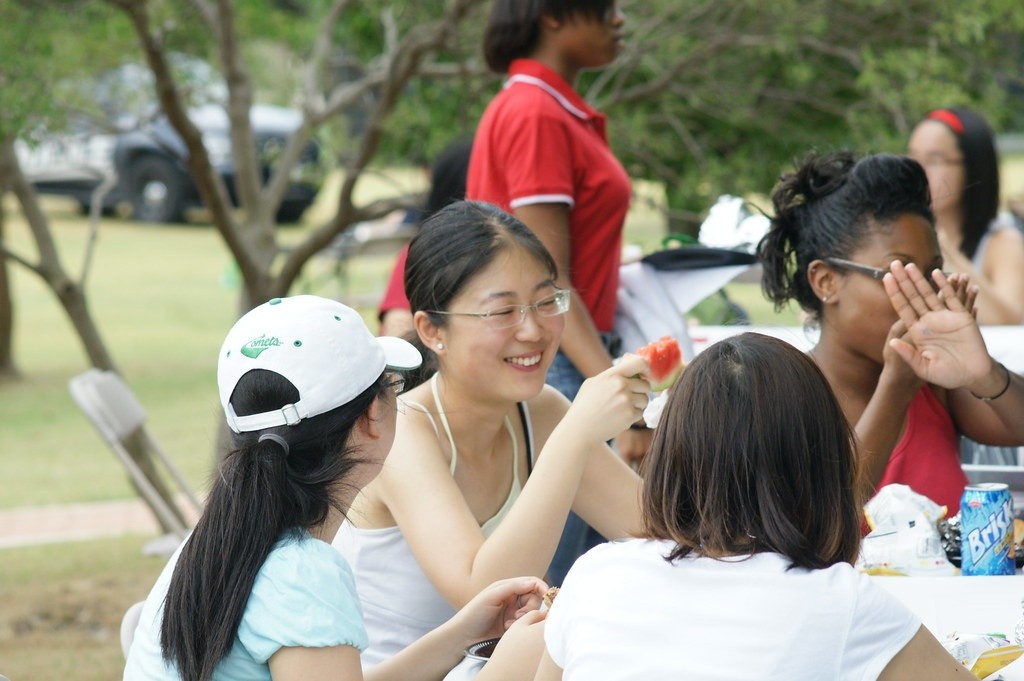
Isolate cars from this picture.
[15,49,322,223]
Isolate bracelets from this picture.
[631,423,647,432]
[970,362,1010,401]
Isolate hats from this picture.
[216,293,422,434]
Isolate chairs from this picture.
[67,367,201,542]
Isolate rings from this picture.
[517,595,522,608]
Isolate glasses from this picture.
[425,286,571,329]
[825,257,952,287]
[379,371,405,394]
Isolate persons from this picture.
[906,108,1024,327]
[466,0,651,587]
[762,153,1024,536]
[377,130,478,336]
[535,332,978,681]
[332,201,645,672]
[122,295,550,681]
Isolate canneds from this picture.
[959,483,1015,577]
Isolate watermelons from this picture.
[613,335,687,392]
